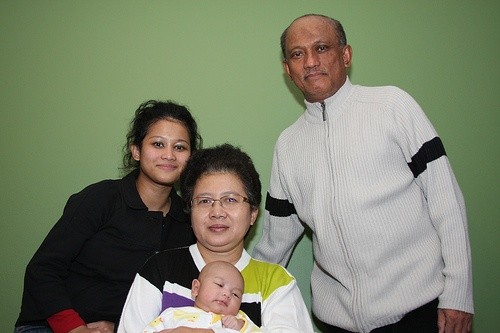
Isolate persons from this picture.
[143,261,259,332]
[115,143,314,333]
[249,14,474,333]
[13,99,202,333]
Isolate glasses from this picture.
[190,195,253,207]
